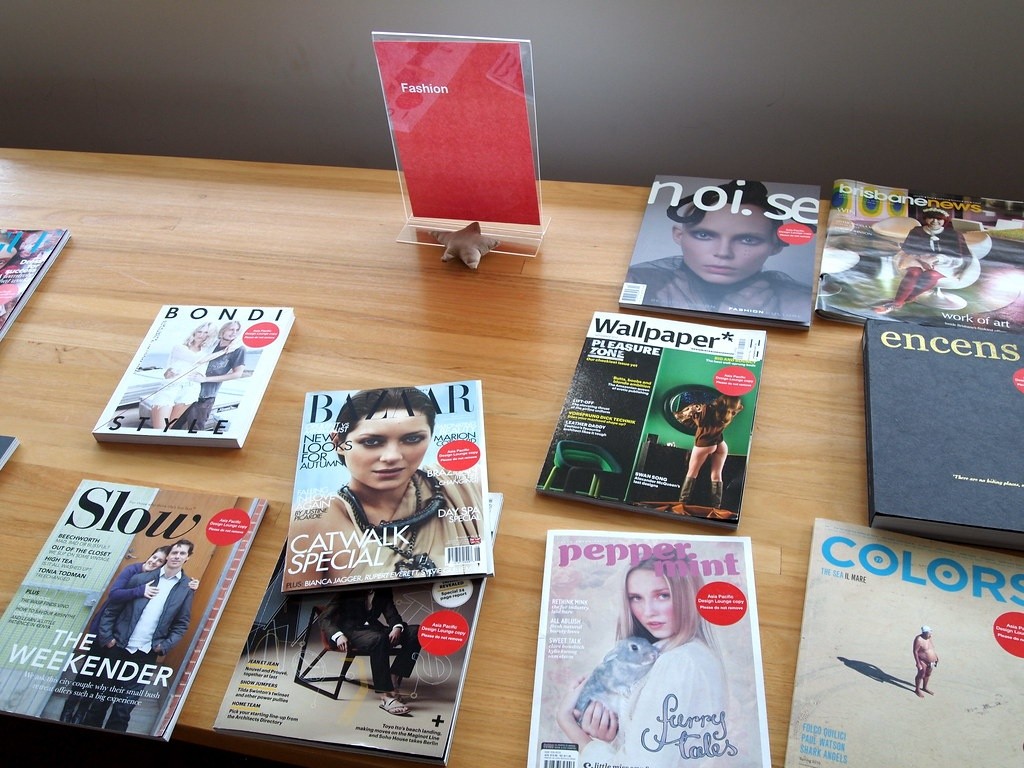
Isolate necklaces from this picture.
[338,474,440,580]
[345,468,443,535]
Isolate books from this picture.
[280,379,497,593]
[535,311,766,528]
[816,175,1024,329]
[783,515,1024,768]
[0,477,270,743]
[212,486,504,763]
[616,175,820,331]
[522,529,775,768]
[0,227,72,343]
[0,436,21,470]
[865,320,1024,552]
[91,304,296,449]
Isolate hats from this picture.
[921,625,932,633]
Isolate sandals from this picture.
[379,697,411,715]
[392,694,406,704]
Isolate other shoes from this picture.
[889,296,917,304]
[72,712,84,724]
[59,703,76,723]
[873,301,902,314]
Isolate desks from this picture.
[0,149,1024,767]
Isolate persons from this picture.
[618,178,812,325]
[555,549,728,768]
[139,319,246,431]
[318,589,422,715]
[284,384,482,589]
[0,241,17,271]
[913,625,939,698]
[59,537,199,732]
[871,207,960,316]
[679,401,729,509]
